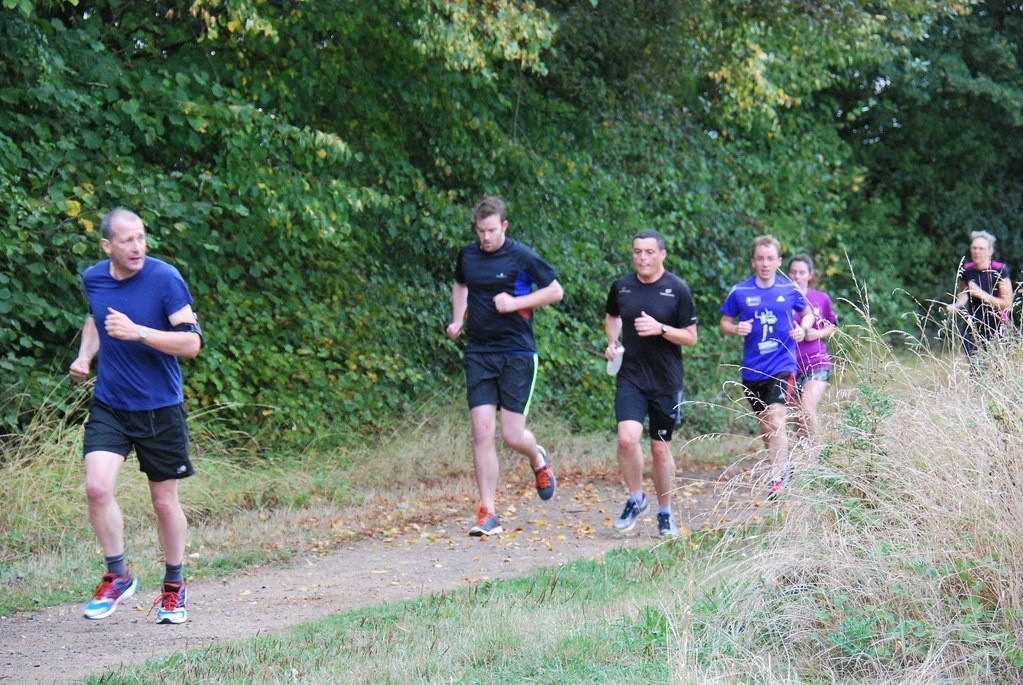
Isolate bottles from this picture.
[606,341,625,376]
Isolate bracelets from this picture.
[800,326,808,338]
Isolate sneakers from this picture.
[469,511,503,537]
[85,567,138,620]
[155,582,188,625]
[768,480,783,500]
[658,512,674,537]
[530,445,556,501]
[616,493,649,533]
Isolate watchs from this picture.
[138,326,148,343]
[659,321,668,337]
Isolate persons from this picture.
[71,207,205,624]
[718,235,813,502]
[448,196,565,537]
[784,253,835,449]
[946,231,1013,386]
[602,230,698,537]
